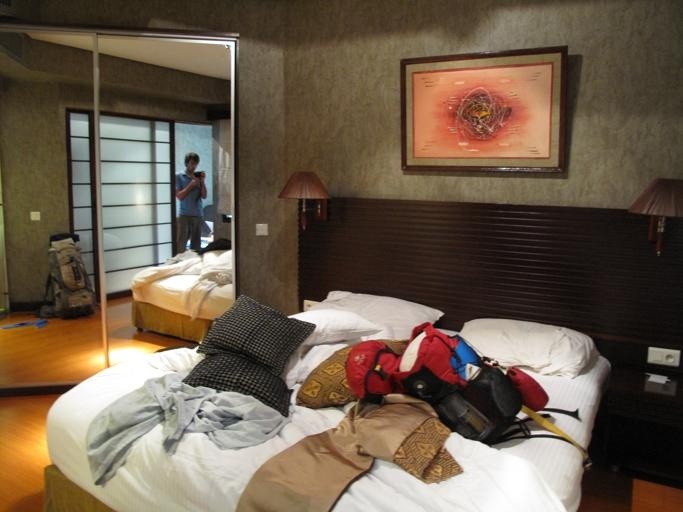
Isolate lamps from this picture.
[276,171,330,232]
[628,174,681,263]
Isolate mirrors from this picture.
[0,22,237,400]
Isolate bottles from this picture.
[439,391,493,441]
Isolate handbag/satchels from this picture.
[345,341,399,403]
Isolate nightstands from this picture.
[602,362,682,492]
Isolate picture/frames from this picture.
[397,42,567,175]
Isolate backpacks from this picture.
[391,322,592,469]
[40,239,96,320]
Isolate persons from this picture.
[175,152,207,253]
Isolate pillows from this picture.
[181,356,295,419]
[196,292,317,379]
[304,290,446,342]
[458,318,596,380]
[293,340,408,411]
[287,305,385,343]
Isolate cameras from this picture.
[194,172,202,177]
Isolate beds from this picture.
[126,247,234,346]
[41,193,640,512]
